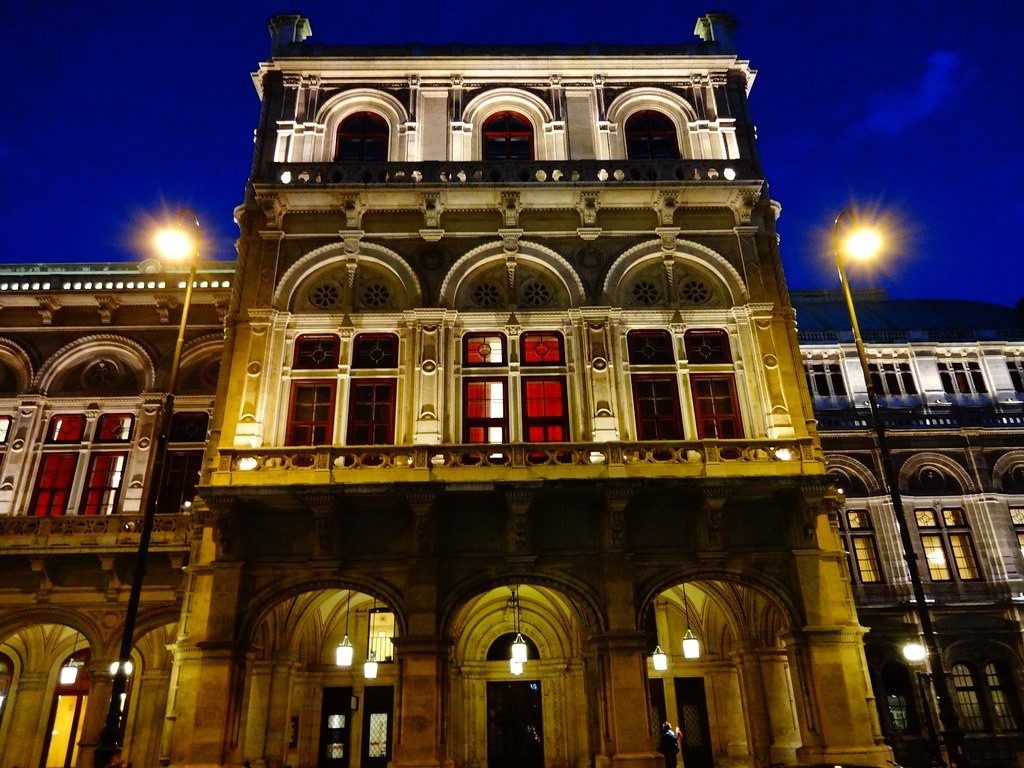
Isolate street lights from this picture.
[89,208,202,768]
[832,204,972,768]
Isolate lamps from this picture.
[510,593,524,676]
[336,590,354,667]
[682,583,700,660]
[652,643,668,671]
[511,584,527,664]
[60,632,79,685]
[363,599,379,680]
